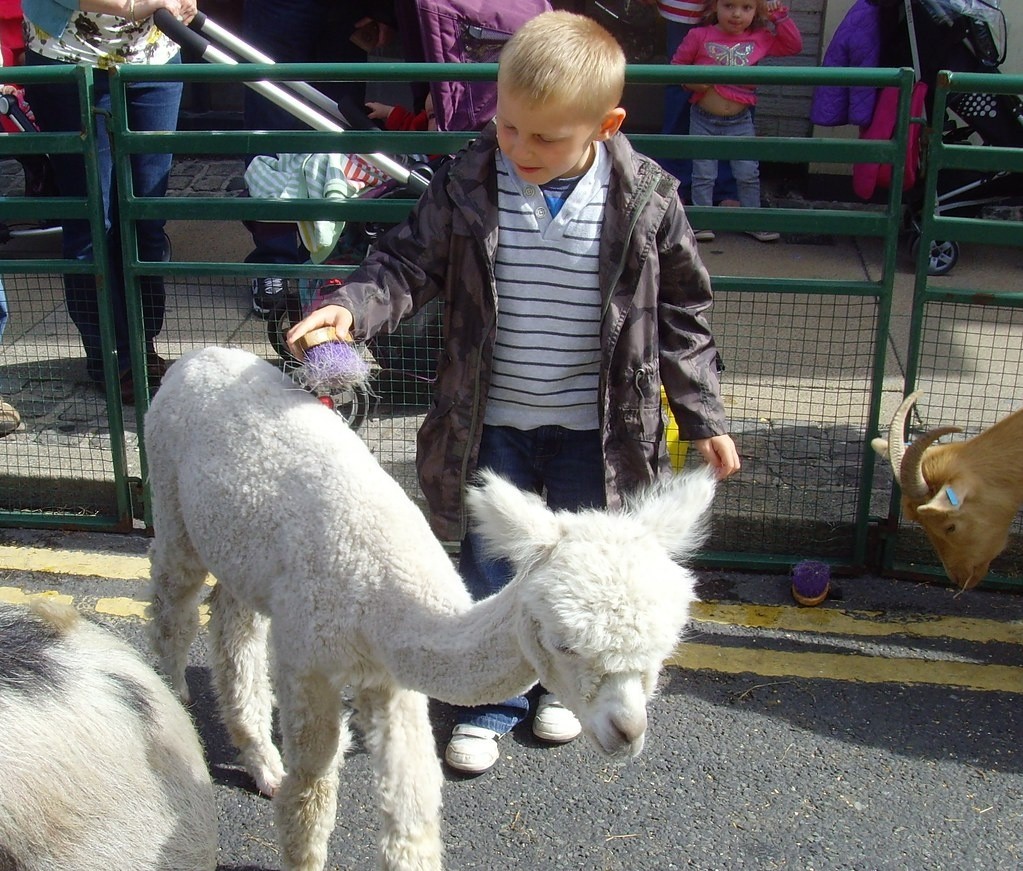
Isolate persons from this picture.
[0,0,803,435]
[286,10,741,773]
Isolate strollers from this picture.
[869,0,1022,273]
[151,5,473,432]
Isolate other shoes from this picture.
[692,229,714,241]
[99,371,136,404]
[147,359,167,386]
[746,227,781,240]
[0,397,21,432]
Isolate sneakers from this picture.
[445,721,502,773]
[533,692,583,743]
[251,272,290,316]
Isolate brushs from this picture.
[299,327,371,387]
[791,560,830,607]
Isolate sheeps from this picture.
[1,587,229,870]
[871,390,1023,593]
